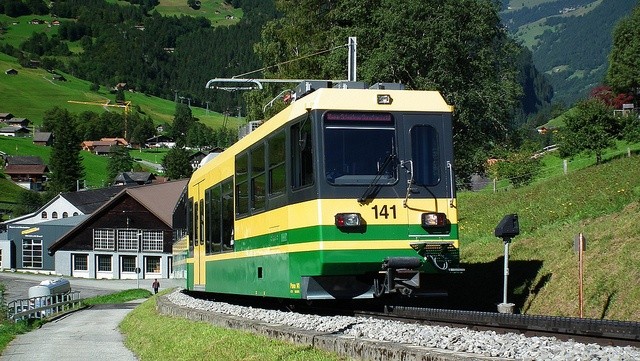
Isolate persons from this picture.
[152,279,159,295]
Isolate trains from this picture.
[172,80,466,313]
